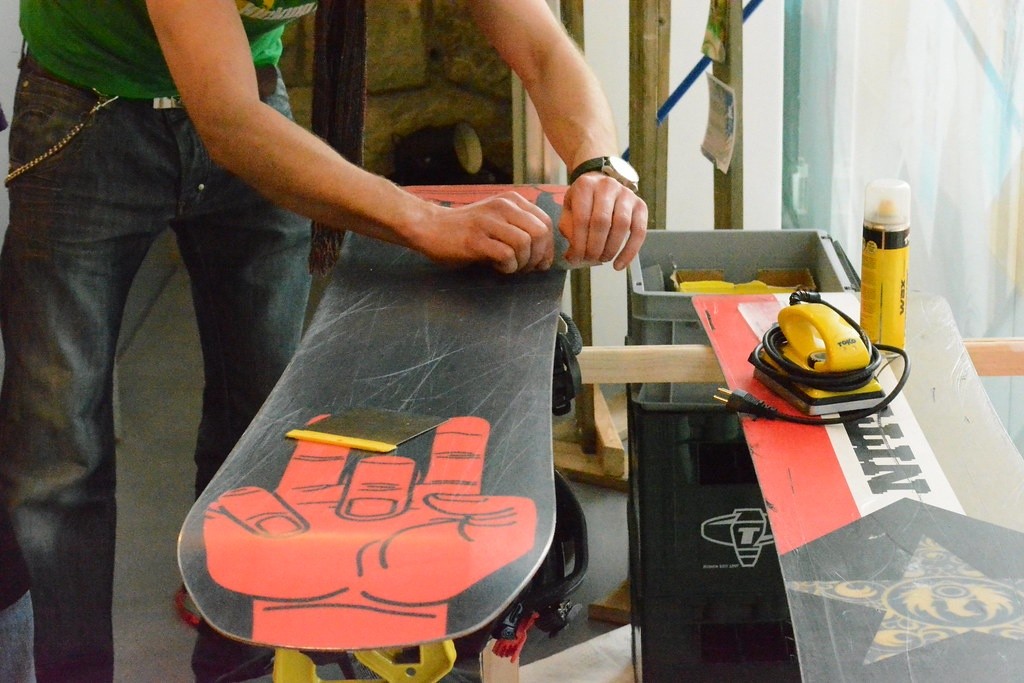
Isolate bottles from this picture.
[859,180,911,360]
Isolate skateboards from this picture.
[177,183,571,652]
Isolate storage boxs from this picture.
[622,229,862,412]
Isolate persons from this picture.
[0,0,649,683]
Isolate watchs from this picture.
[569,155,641,194]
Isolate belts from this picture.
[24,46,278,108]
